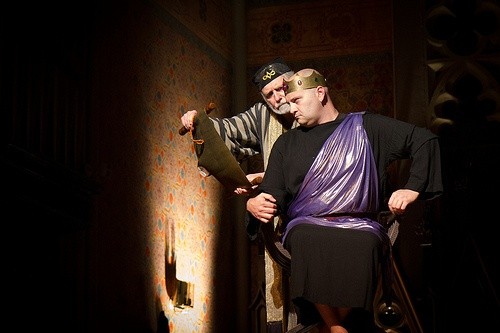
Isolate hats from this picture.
[249,56,292,93]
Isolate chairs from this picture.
[263,196,423,333]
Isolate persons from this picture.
[245,67,442,333]
[180,65,296,331]
